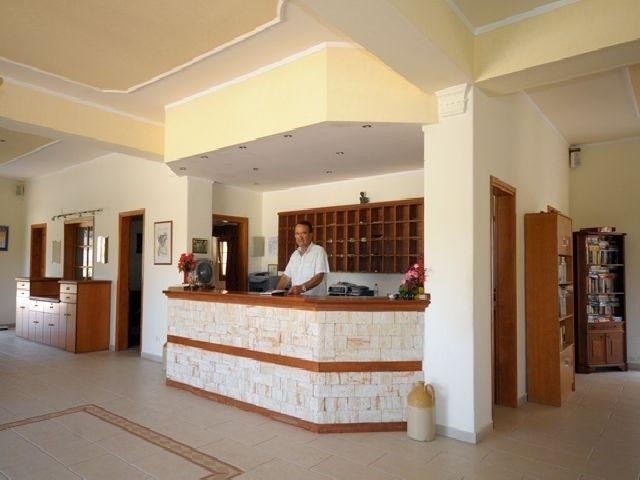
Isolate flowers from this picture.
[400,256,424,300]
[178,254,196,271]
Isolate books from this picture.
[561,324,565,351]
[588,266,617,292]
[558,285,567,317]
[588,295,622,322]
[587,235,618,264]
[557,256,566,282]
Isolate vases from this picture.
[184,273,189,283]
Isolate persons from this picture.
[274,220,330,296]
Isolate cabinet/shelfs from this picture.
[525,211,574,404]
[573,230,628,374]
[28,296,60,347]
[59,281,110,352]
[15,277,62,337]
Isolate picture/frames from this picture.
[0,225,8,251]
[154,221,173,265]
[191,238,209,259]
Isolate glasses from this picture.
[295,232,310,235]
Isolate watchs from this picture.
[301,284,306,291]
